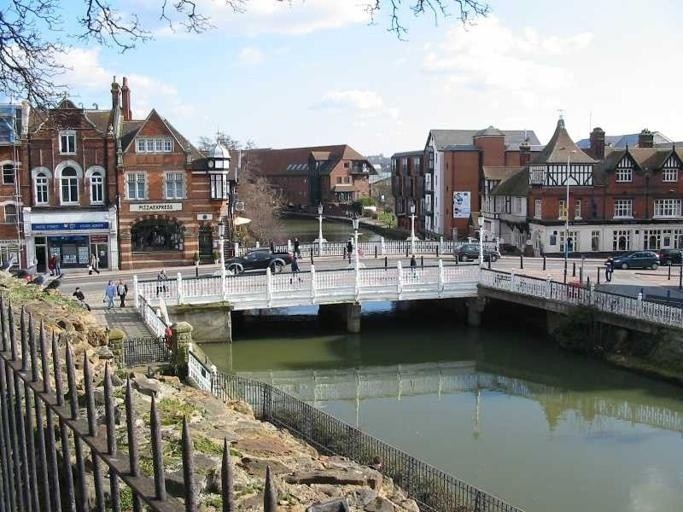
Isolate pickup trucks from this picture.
[223,248,295,273]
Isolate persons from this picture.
[293,237,303,259]
[115,279,128,308]
[604,255,614,282]
[156,268,169,297]
[103,279,117,309]
[87,253,100,275]
[48,255,55,276]
[54,253,60,276]
[345,242,353,264]
[410,254,418,279]
[288,257,303,285]
[72,287,85,302]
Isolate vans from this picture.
[602,251,663,272]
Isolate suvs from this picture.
[656,247,683,267]
[453,243,501,262]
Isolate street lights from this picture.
[345,210,365,296]
[472,387,481,442]
[408,199,420,253]
[564,147,579,278]
[312,201,329,257]
[475,211,486,269]
